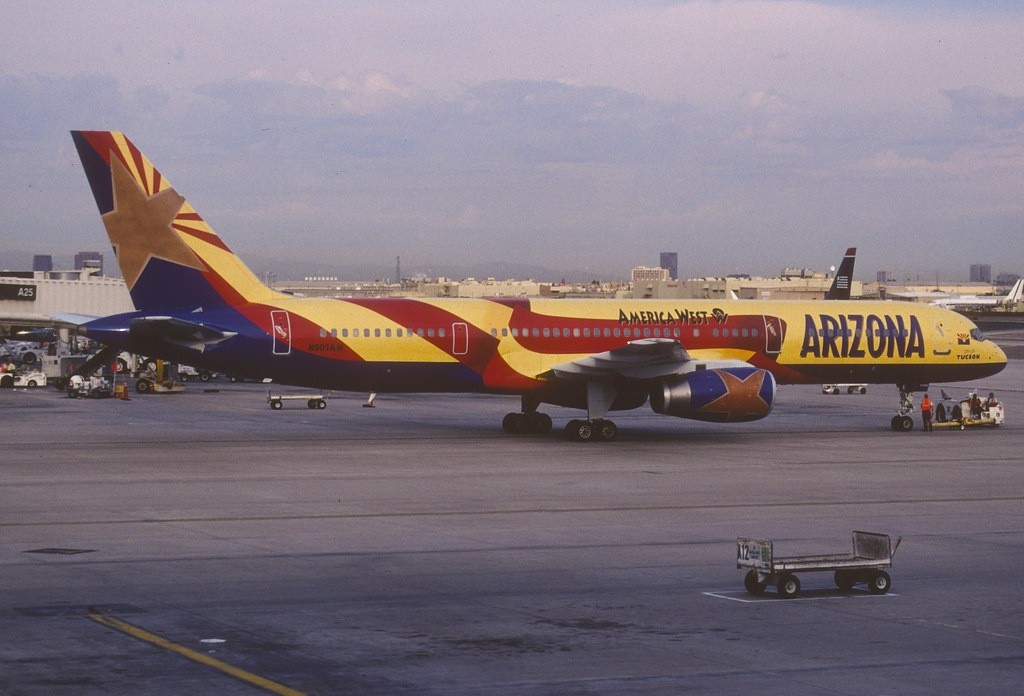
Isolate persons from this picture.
[921,393,934,432]
[969,394,984,419]
[985,392,998,411]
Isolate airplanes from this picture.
[45,126,1015,443]
[924,278,1024,316]
